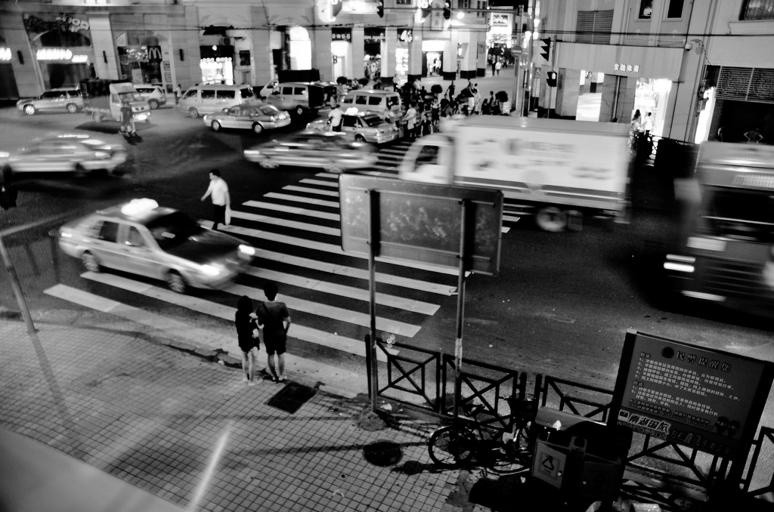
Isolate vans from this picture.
[175,84,256,120]
[265,82,323,116]
[337,89,401,122]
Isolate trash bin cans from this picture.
[528,406,633,502]
[654,136,700,182]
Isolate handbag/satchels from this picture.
[225,205,232,225]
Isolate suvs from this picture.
[132,84,166,110]
[15,87,83,116]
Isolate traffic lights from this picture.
[0,163,18,212]
[546,72,556,87]
[540,37,551,61]
[442,0,452,20]
[376,1,384,19]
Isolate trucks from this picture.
[660,141,773,322]
[78,78,151,126]
[259,69,320,101]
[397,115,633,234]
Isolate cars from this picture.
[202,99,291,135]
[242,128,376,175]
[0,129,127,188]
[305,107,399,144]
[57,198,256,294]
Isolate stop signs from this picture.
[511,45,522,58]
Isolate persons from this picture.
[716,126,728,142]
[392,72,482,140]
[255,282,291,381]
[120,100,136,134]
[491,55,507,76]
[175,83,182,104]
[632,109,641,127]
[743,127,763,144]
[365,58,381,78]
[486,90,495,116]
[338,76,360,103]
[234,294,264,386]
[199,168,230,231]
[328,104,343,131]
[642,111,652,135]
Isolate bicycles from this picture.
[427,392,538,473]
